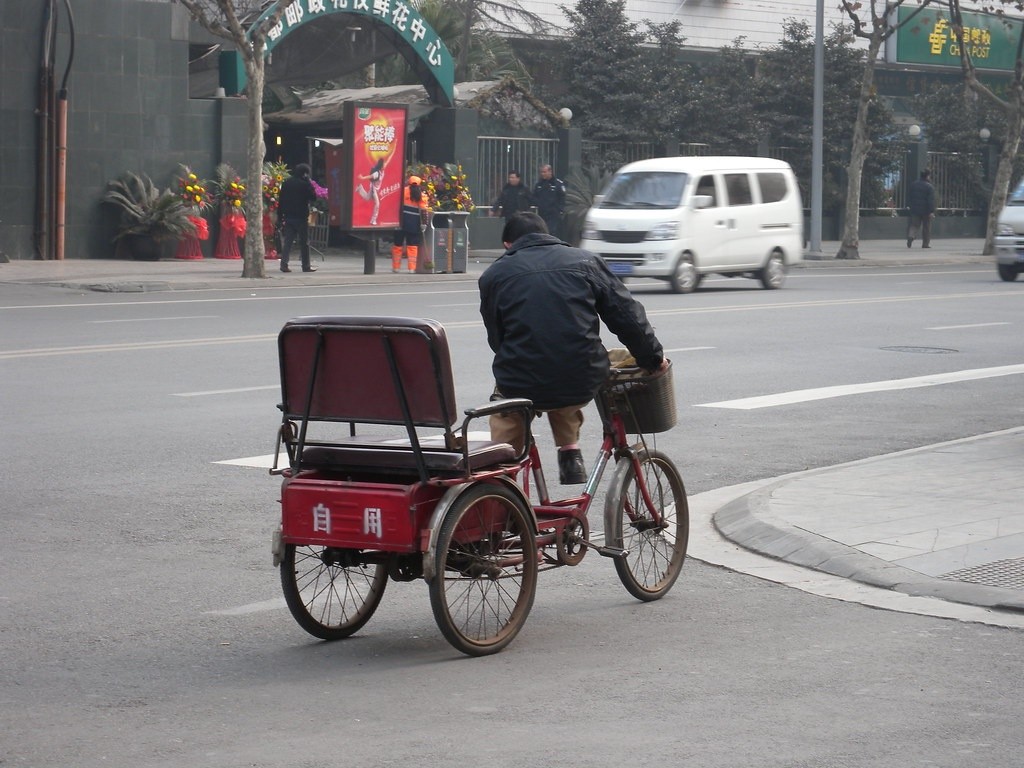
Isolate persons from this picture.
[532,165,566,238]
[392,175,428,274]
[489,171,532,224]
[355,139,398,225]
[275,163,317,273]
[906,169,935,248]
[478,212,667,485]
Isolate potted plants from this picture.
[101,169,200,261]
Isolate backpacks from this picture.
[369,168,380,182]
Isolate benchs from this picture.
[277,316,515,471]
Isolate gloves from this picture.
[420,224,427,233]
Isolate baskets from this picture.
[594,358,677,434]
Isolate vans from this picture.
[580,155,804,292]
[993,175,1024,281]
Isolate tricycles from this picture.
[269,315,690,657]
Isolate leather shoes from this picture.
[558,449,587,484]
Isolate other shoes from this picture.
[921,245,932,248]
[280,267,291,272]
[392,268,399,273]
[409,270,417,274]
[355,184,362,192]
[370,221,377,225]
[907,237,913,248]
[303,268,317,272]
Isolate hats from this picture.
[410,176,421,185]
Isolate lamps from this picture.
[979,127,991,143]
[558,107,574,127]
[908,125,921,141]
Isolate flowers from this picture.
[211,162,246,207]
[311,180,329,199]
[259,160,291,208]
[404,161,476,214]
[174,161,217,212]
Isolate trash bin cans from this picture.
[415,211,470,274]
